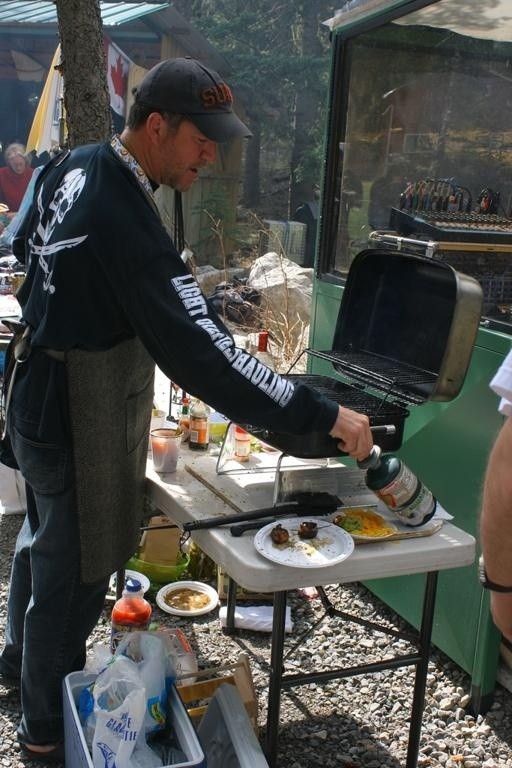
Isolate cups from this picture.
[149,426,184,472]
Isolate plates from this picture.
[104,568,151,600]
[327,511,398,542]
[253,516,357,570]
[156,579,220,617]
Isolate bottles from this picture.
[252,331,277,376]
[358,444,437,527]
[234,426,250,461]
[110,578,152,659]
[189,399,210,451]
[178,397,191,441]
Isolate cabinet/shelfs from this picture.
[364,228,512,331]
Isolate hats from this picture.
[134,58,253,144]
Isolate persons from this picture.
[0,54,375,761]
[480,347,512,667]
[1,142,34,223]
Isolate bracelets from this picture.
[478,553,511,593]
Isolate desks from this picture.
[121,366,481,762]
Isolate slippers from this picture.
[19,740,65,762]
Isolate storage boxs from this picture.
[59,668,208,767]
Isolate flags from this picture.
[106,38,131,117]
